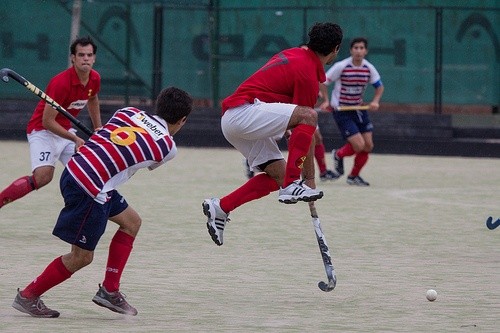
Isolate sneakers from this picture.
[202,198,230,246]
[92,284,137,316]
[244,158,254,179]
[278,179,324,204]
[333,149,344,175]
[12,288,60,318]
[346,176,370,187]
[319,169,340,182]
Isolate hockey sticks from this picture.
[308,200,337,293]
[486,216,500,231]
[313,105,371,113]
[0,67,93,137]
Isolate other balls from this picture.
[425,289,438,302]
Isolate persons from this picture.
[319,38,384,187]
[202,22,343,246]
[0,37,103,208]
[12,87,192,318]
